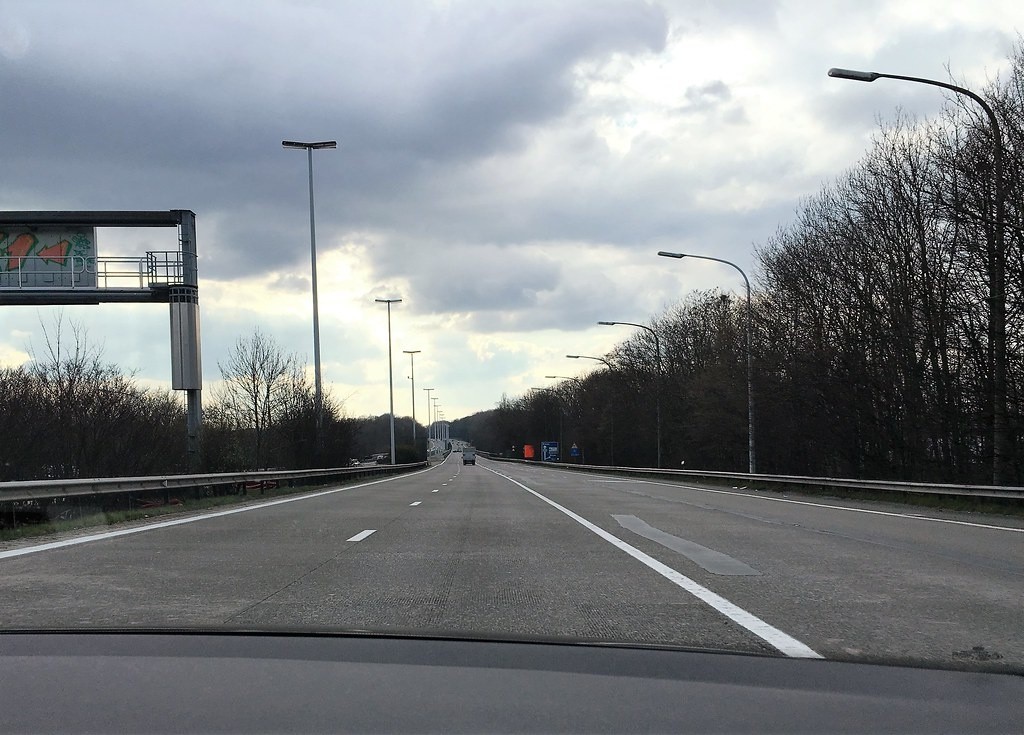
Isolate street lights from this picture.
[402,349,421,440]
[566,354,614,465]
[375,296,403,465]
[597,321,662,469]
[658,249,758,472]
[422,387,448,465]
[828,66,1012,485]
[530,375,587,465]
[280,133,339,466]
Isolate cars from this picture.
[346,451,390,466]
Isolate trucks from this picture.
[461,447,478,465]
